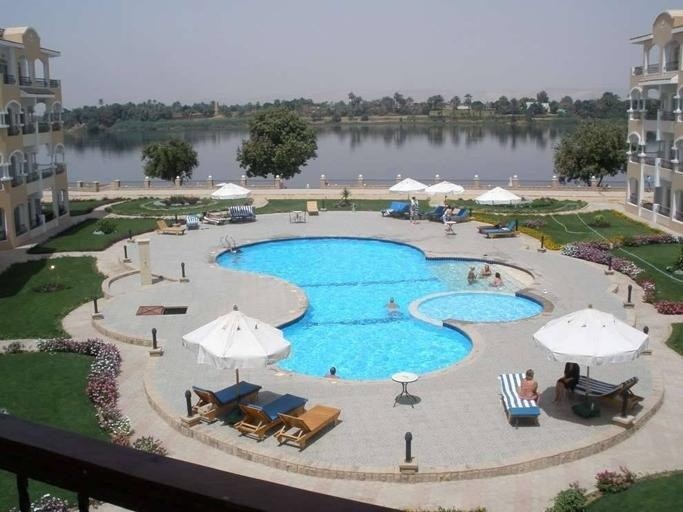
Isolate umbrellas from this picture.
[426,180,465,206]
[387,177,428,201]
[180,303,292,424]
[209,182,251,207]
[473,186,521,229]
[530,307,650,419]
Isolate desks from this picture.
[290,210,305,224]
[389,372,420,409]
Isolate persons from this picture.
[438,203,455,226]
[198,209,225,226]
[551,362,579,407]
[490,272,502,288]
[408,196,419,224]
[517,368,540,403]
[479,265,491,275]
[382,297,401,312]
[467,265,478,285]
[324,367,341,379]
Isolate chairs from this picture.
[498,373,541,430]
[379,200,518,240]
[153,203,256,236]
[188,377,341,452]
[559,374,644,419]
[306,200,318,216]
